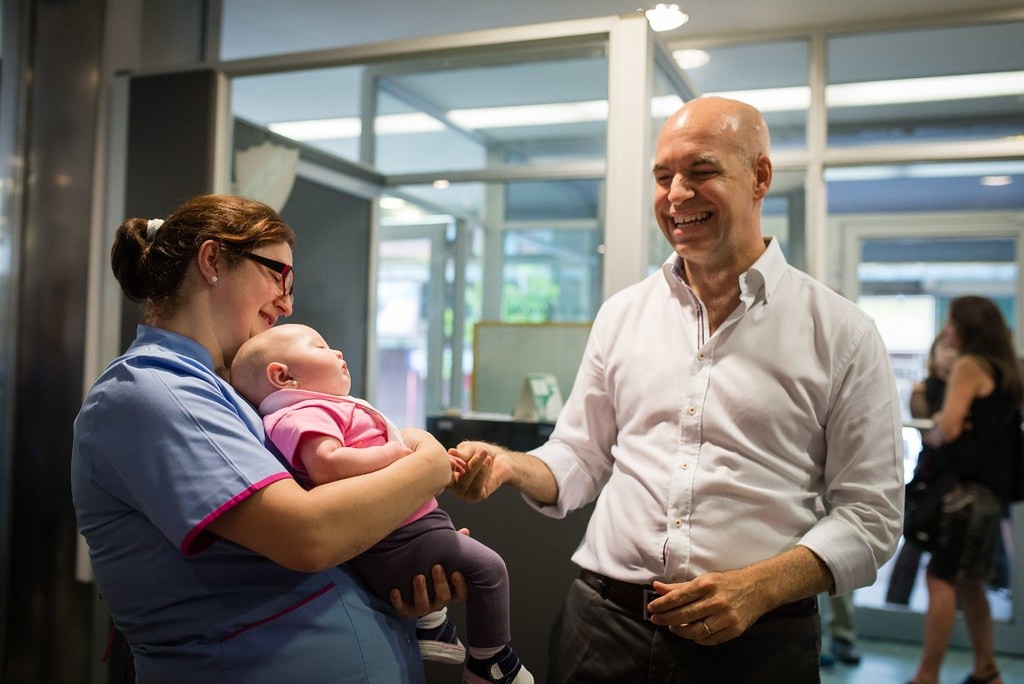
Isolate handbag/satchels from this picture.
[904,471,972,556]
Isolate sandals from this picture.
[962,671,1003,684]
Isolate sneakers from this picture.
[462,645,535,684]
[415,615,466,664]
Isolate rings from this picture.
[701,620,712,636]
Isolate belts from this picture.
[579,569,818,633]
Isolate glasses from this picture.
[223,238,295,297]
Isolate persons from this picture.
[228,324,534,684]
[442,96,905,684]
[905,293,1024,684]
[883,332,968,613]
[68,193,470,684]
